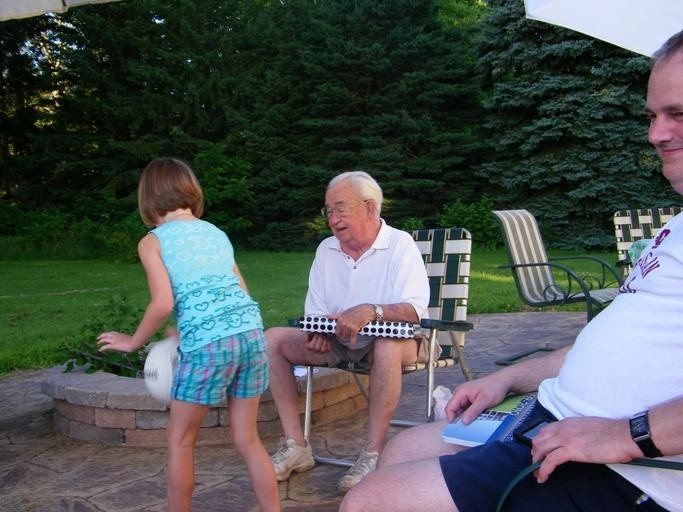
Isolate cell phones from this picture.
[513,414,553,448]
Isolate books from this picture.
[442,389,540,448]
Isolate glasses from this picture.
[321,199,366,218]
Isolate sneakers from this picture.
[336,447,379,493]
[270,436,315,481]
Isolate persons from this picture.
[265,170,443,491]
[96,158,282,511]
[341,31,682,512]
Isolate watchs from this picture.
[370,302,384,323]
[630,410,662,459]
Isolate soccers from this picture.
[144,336,181,407]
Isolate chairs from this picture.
[490,208,623,365]
[613,208,683,282]
[493,458,682,511]
[288,227,472,468]
